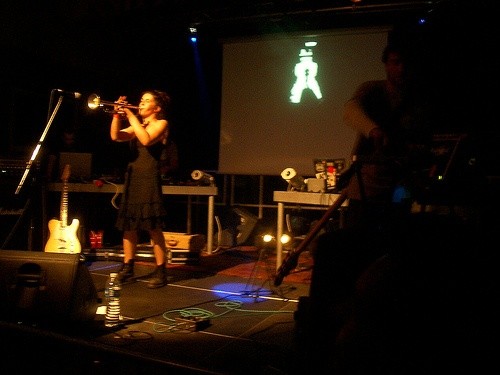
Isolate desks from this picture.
[42,183,219,256]
[273,191,432,273]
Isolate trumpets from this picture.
[87,94,143,120]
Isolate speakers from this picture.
[0,250,97,334]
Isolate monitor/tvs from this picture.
[60,153,92,179]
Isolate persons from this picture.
[156,141,179,225]
[43,129,86,218]
[344,40,430,238]
[110,90,173,289]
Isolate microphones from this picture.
[53,88,81,99]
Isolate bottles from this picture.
[104,272,122,326]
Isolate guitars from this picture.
[44,164,81,255]
[271,140,391,288]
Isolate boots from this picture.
[115,263,135,286]
[148,265,167,288]
